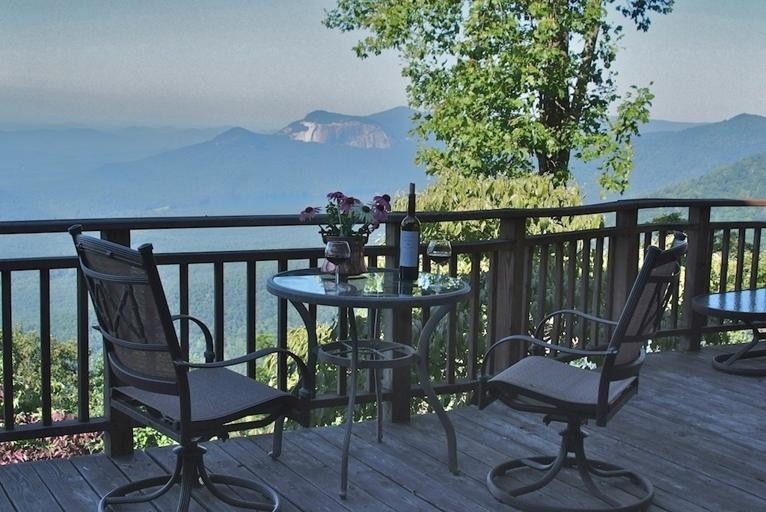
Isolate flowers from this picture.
[299,191,392,273]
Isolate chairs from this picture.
[474,229,690,512]
[68,223,313,512]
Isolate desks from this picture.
[691,286,766,377]
[266,267,473,500]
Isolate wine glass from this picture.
[427,240,453,291]
[324,241,350,293]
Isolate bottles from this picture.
[399,182,421,281]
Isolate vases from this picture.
[321,235,368,276]
